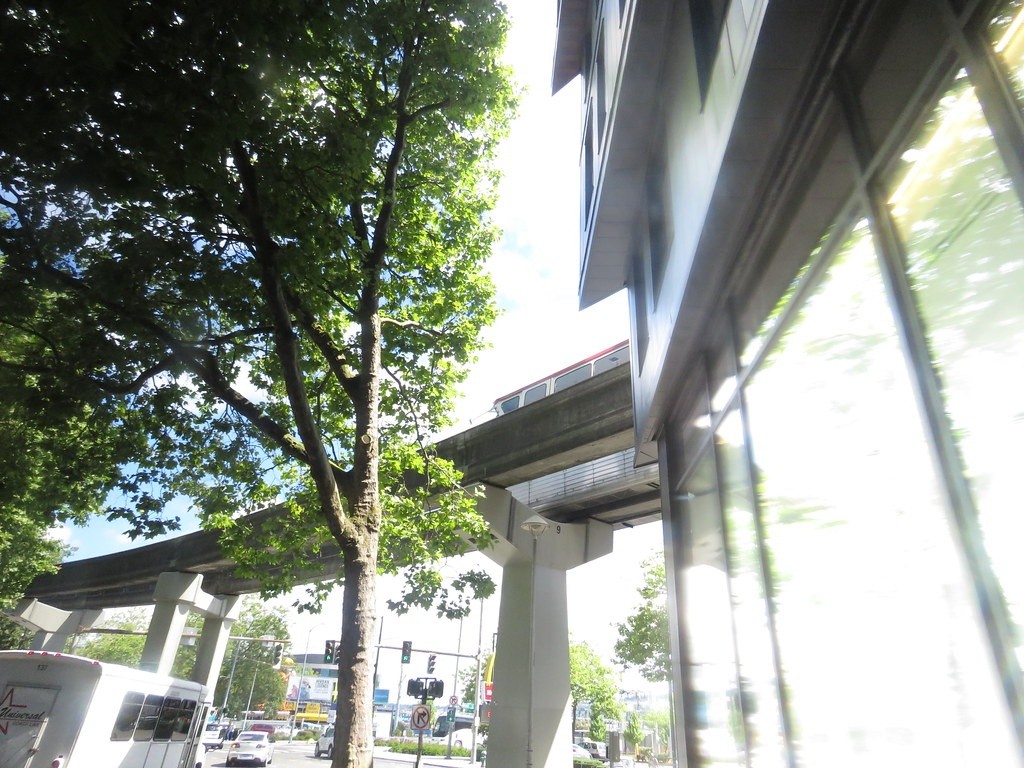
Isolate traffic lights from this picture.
[335,644,342,665]
[426,654,437,675]
[324,639,335,664]
[401,641,413,664]
[274,644,283,666]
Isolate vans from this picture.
[578,742,607,763]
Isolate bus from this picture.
[2,650,210,768]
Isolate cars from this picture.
[249,724,274,735]
[226,731,274,767]
[203,722,224,753]
[572,744,594,761]
[314,725,334,758]
